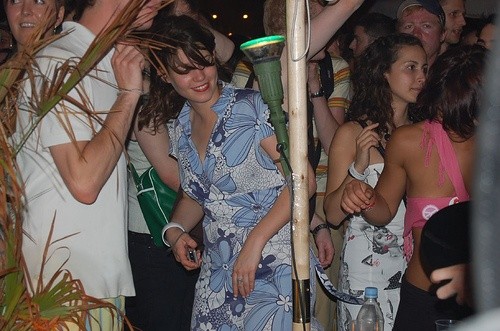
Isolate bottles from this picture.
[357,287,384,331]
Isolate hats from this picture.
[396,0,446,29]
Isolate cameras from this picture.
[186,248,198,264]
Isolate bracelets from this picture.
[161,223,185,247]
[347,169,355,178]
[312,224,331,236]
[117,89,143,96]
[310,92,324,97]
[168,232,188,252]
[360,200,376,211]
[348,161,370,181]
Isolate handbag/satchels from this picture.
[136,166,179,250]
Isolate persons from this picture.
[0,0,500,331]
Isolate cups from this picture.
[435,319,462,331]
[349,320,378,331]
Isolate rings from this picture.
[238,280,242,283]
